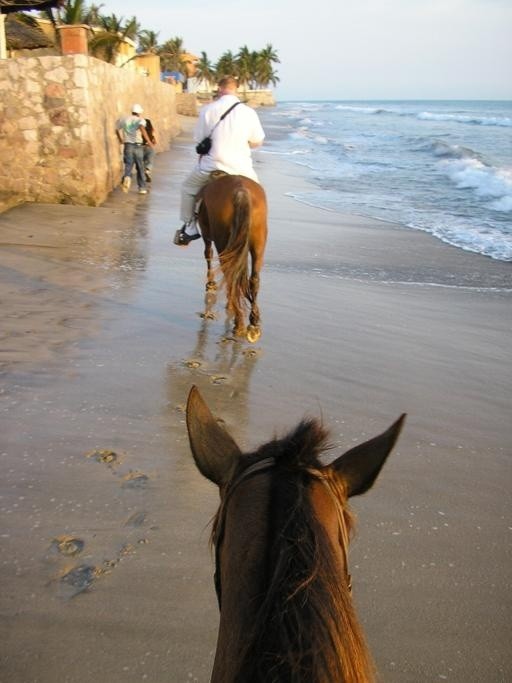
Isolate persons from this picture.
[174,75,266,246]
[141,117,158,182]
[115,103,156,194]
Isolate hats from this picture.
[131,103,143,114]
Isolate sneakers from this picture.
[137,187,147,194]
[179,223,201,241]
[121,176,130,193]
[145,169,151,183]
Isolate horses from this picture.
[185,380,409,683]
[193,169,270,344]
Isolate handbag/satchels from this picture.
[196,137,211,155]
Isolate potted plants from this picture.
[56,0,161,80]
